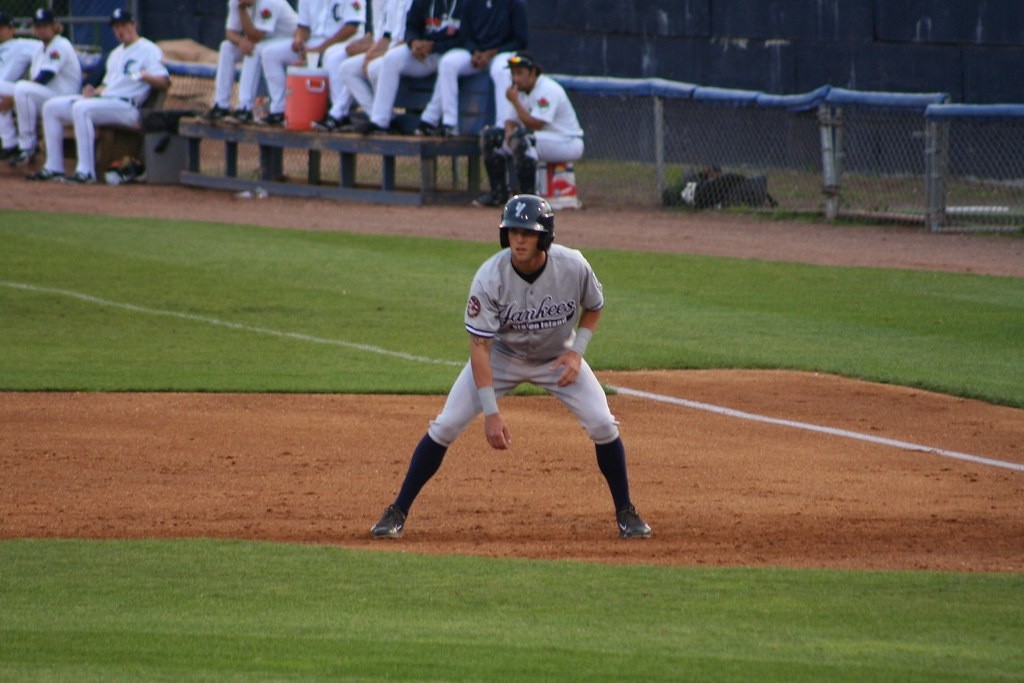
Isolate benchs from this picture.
[13,77,168,182]
[178,65,496,206]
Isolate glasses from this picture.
[509,57,533,67]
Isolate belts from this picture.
[93,94,140,109]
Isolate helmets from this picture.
[498,194,555,250]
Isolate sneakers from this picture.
[0,146,18,159]
[9,146,39,168]
[371,505,406,539]
[473,192,513,209]
[349,121,388,135]
[201,104,228,120]
[432,125,460,137]
[223,109,254,127]
[311,117,352,131]
[65,173,96,184]
[26,168,66,182]
[414,121,436,137]
[616,504,651,538]
[257,112,283,127]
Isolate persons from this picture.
[370,195,653,540]
[26,8,169,182]
[471,52,585,209]
[202,0,299,124]
[0,7,82,168]
[311,0,528,139]
[258,0,366,131]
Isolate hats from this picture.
[0,14,15,26]
[107,7,134,27]
[31,7,54,24]
[504,53,535,70]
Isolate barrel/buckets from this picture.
[284,65,329,130]
